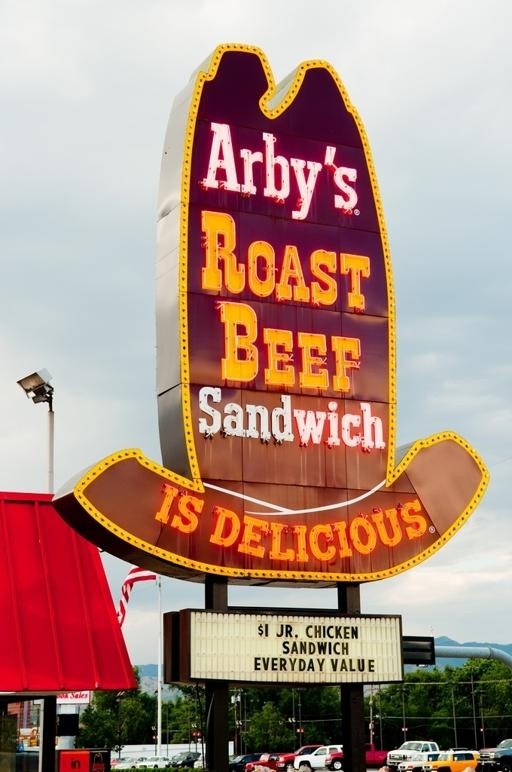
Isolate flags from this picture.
[116,566,156,626]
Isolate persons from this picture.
[246,763,277,772]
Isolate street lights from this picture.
[17,369,55,501]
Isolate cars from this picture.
[110,751,206,768]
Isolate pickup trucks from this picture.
[229,742,388,772]
[387,740,512,772]
